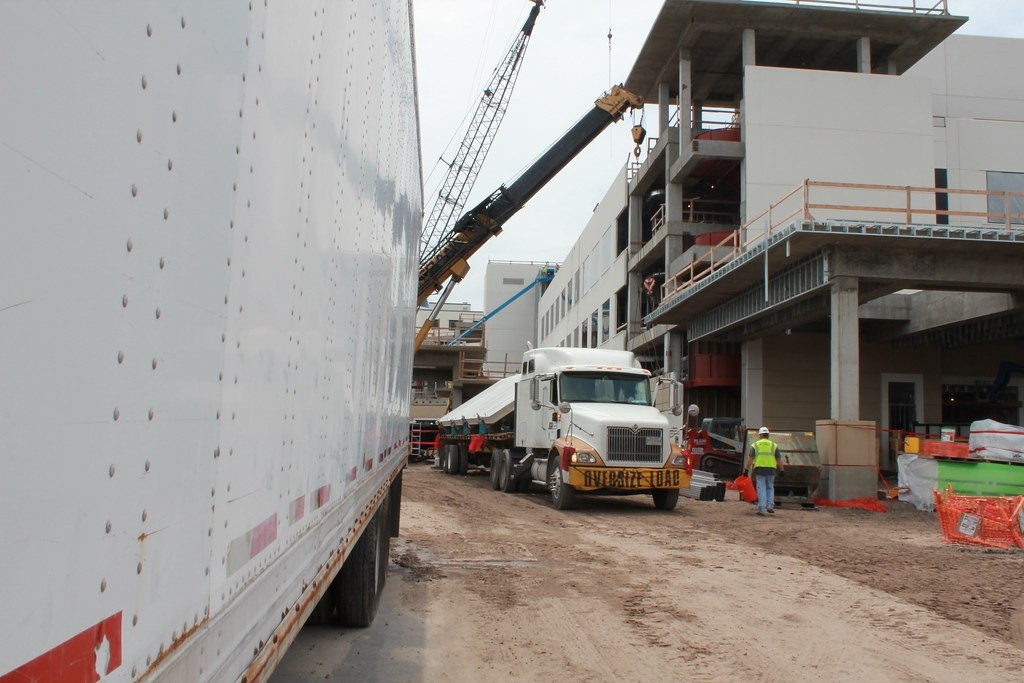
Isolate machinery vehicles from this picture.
[688,416,745,481]
[442,340,700,512]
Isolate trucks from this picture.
[1,1,408,683]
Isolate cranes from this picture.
[408,0,647,461]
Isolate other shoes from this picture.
[757,508,775,516]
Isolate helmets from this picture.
[758,426,769,434]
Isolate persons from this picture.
[744,427,784,516]
